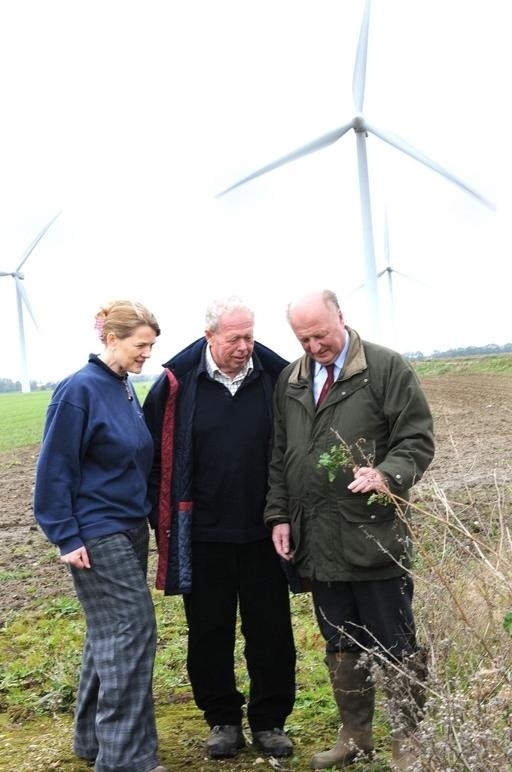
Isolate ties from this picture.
[315,363,334,413]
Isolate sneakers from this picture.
[206,725,245,759]
[252,727,293,759]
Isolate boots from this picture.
[384,647,427,772]
[309,652,375,770]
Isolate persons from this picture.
[30,298,165,772]
[261,285,439,770]
[141,298,300,760]
